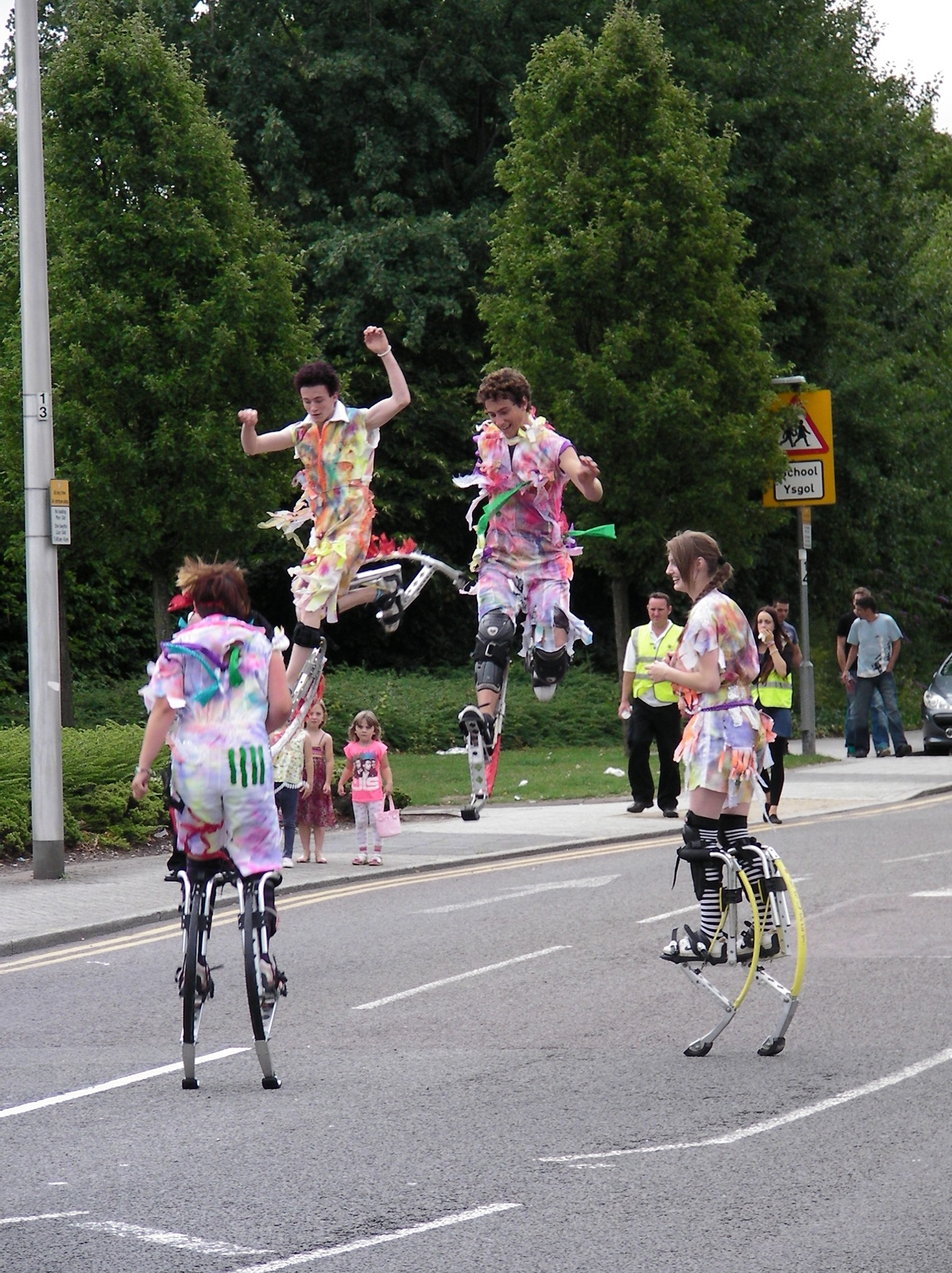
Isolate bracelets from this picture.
[769,649,778,655]
[377,344,392,358]
[765,641,774,647]
[134,766,156,777]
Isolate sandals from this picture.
[352,854,367,864]
[369,855,382,865]
[376,577,400,632]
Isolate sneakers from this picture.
[663,934,722,958]
[736,933,772,954]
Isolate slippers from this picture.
[297,857,309,862]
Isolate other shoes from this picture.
[856,751,866,757]
[180,966,208,992]
[895,744,912,756]
[459,705,493,755]
[283,857,293,867]
[770,814,782,824]
[316,858,326,863]
[763,803,770,822]
[260,960,283,989]
[531,670,556,701]
[878,750,890,757]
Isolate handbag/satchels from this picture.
[375,793,401,837]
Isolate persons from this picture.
[618,592,684,819]
[266,712,314,869]
[295,699,334,864]
[836,586,912,758]
[773,592,802,756]
[338,709,393,864]
[645,529,781,960]
[236,324,411,693]
[458,369,604,757]
[750,605,794,826]
[132,548,293,992]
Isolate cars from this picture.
[920,652,952,754]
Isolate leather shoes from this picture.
[626,801,652,812]
[663,807,678,817]
